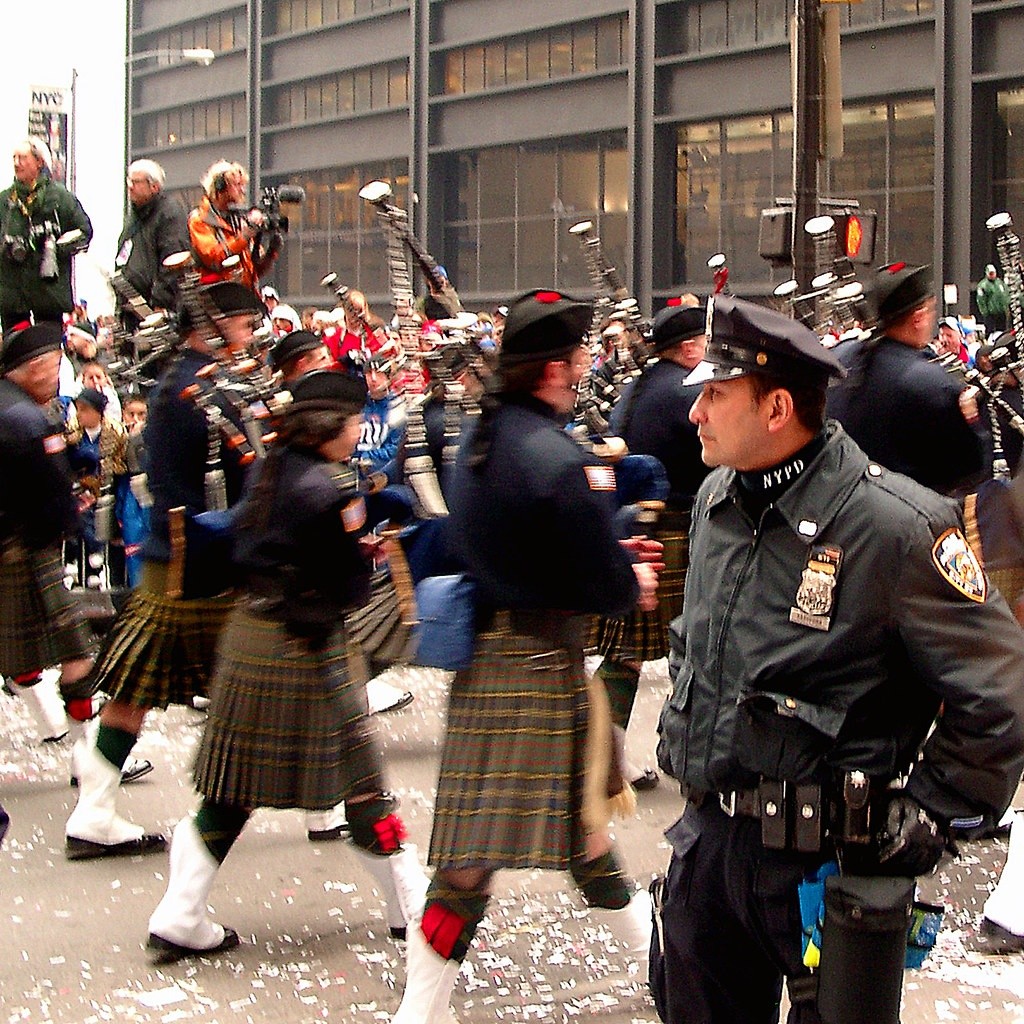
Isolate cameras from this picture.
[29,221,62,282]
[0,234,27,263]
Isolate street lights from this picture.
[66,47,214,306]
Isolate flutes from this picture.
[771,211,877,349]
[571,215,657,360]
[358,179,492,516]
[982,206,1023,379]
[97,179,1024,514]
[185,379,263,463]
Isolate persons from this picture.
[113,158,190,362]
[0,136,93,340]
[146,370,431,959]
[183,160,283,350]
[2,265,1024,947]
[654,292,1024,1023]
[388,289,664,1024]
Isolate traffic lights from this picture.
[842,209,877,263]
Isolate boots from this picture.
[590,890,655,988]
[65,746,165,861]
[8,678,105,744]
[967,807,1024,950]
[304,798,350,838]
[390,914,460,1024]
[345,838,433,939]
[66,711,153,787]
[145,817,241,955]
[365,679,413,711]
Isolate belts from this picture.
[680,782,890,844]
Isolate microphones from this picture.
[277,185,304,203]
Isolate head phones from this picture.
[214,171,228,192]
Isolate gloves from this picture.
[864,789,945,883]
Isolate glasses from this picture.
[125,178,147,188]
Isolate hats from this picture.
[500,289,595,355]
[868,261,937,320]
[0,322,59,373]
[284,370,370,406]
[651,300,708,350]
[77,388,110,415]
[985,265,996,273]
[268,329,323,367]
[364,358,394,378]
[683,295,845,390]
[937,316,960,330]
[183,280,268,326]
